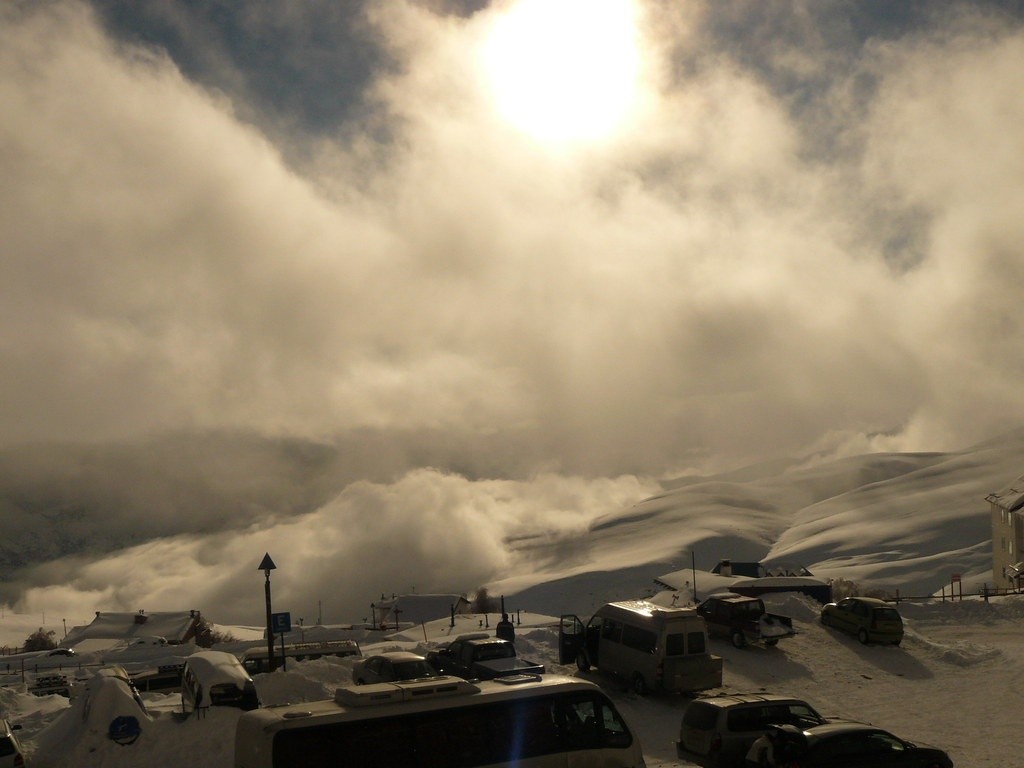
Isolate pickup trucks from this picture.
[425,633,546,683]
[695,592,799,650]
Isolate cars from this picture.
[67,664,152,745]
[0,717,26,768]
[764,720,953,768]
[351,651,441,689]
[821,597,905,648]
[48,647,75,657]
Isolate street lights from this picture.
[259,552,277,671]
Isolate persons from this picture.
[496,613,515,644]
[7,664,10,673]
[746,733,776,766]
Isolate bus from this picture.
[239,639,362,677]
[232,671,647,768]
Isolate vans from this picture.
[675,694,859,768]
[178,648,261,715]
[557,599,723,698]
